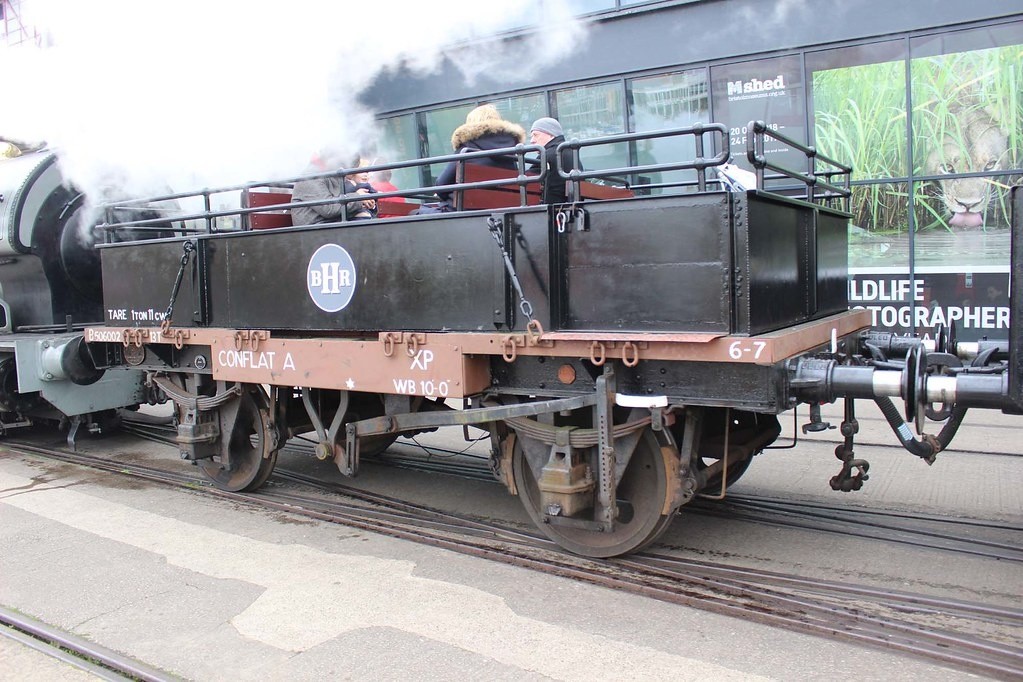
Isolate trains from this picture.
[2,121,1023,565]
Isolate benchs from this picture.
[453,147,635,212]
[240,180,452,231]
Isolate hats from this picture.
[530,118,563,137]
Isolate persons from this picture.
[342,160,378,220]
[408,104,523,215]
[366,158,406,218]
[526,118,584,203]
[291,145,376,226]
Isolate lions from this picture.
[921,70,1008,228]
